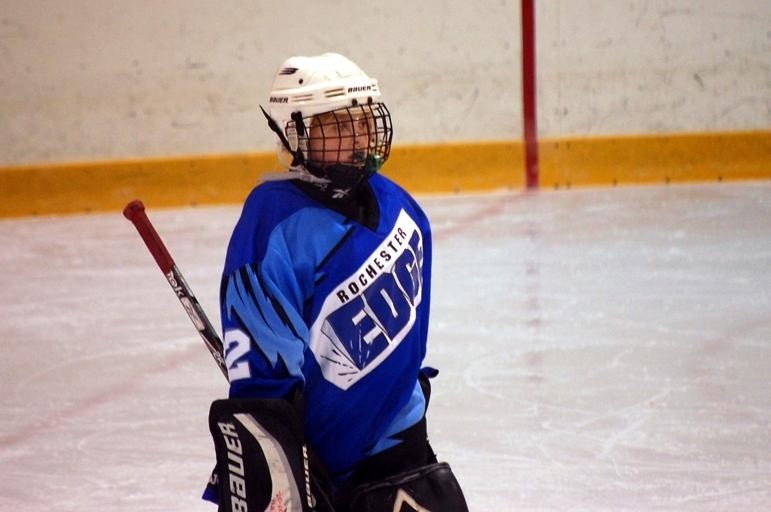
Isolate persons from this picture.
[199,52,470,511]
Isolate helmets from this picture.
[270,52,395,198]
